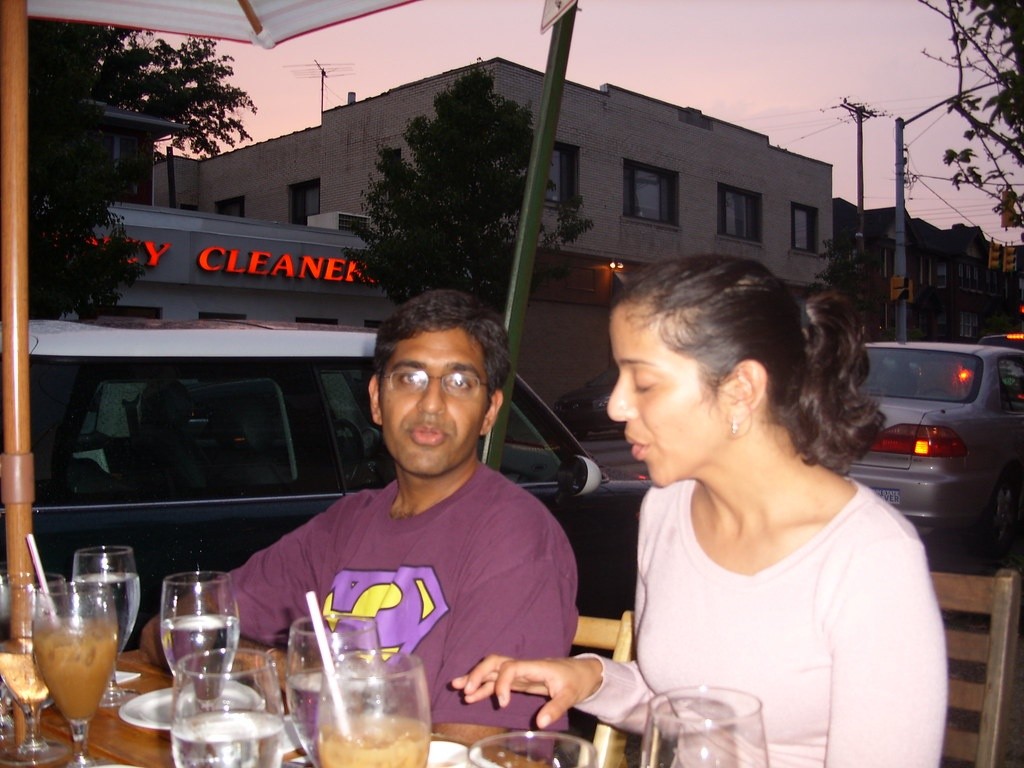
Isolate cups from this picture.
[284,616,377,768]
[640,686,768,768]
[161,571,283,768]
[468,730,600,768]
[312,650,432,768]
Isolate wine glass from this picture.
[73,544,140,708]
[0,571,72,765]
[32,581,119,768]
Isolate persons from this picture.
[449,255,948,768]
[140,292,579,735]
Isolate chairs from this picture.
[133,377,215,501]
[574,607,633,768]
[930,568,1022,768]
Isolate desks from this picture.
[1,640,564,767]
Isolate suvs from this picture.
[0,317,655,713]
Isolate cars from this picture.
[818,339,1024,562]
[953,333,1024,412]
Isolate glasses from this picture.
[379,371,491,399]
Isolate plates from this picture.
[118,686,171,731]
[428,740,470,768]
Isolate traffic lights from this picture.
[1003,246,1016,272]
[988,241,1003,269]
[889,276,909,302]
